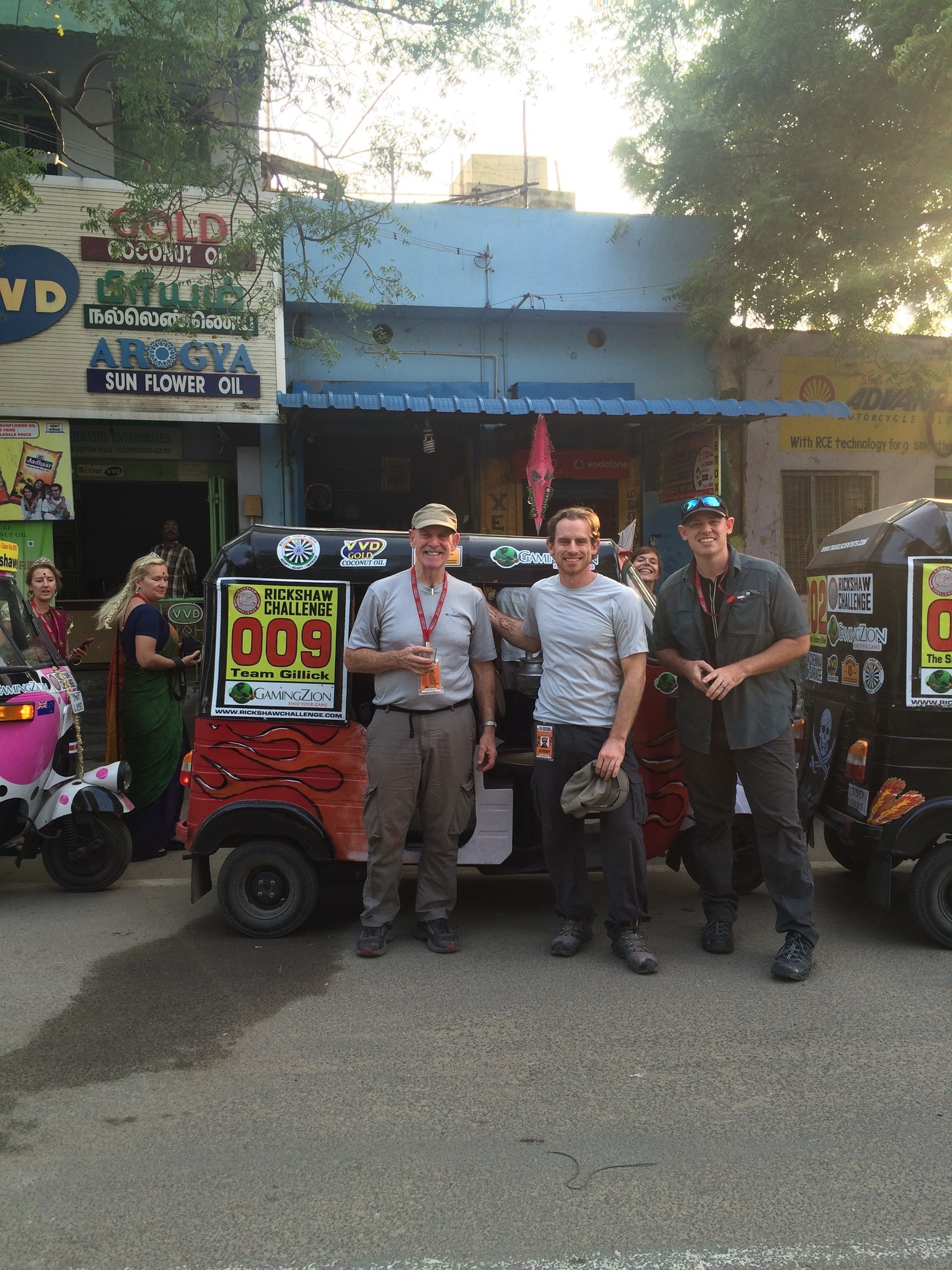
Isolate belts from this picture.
[376,699,468,739]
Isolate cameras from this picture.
[183,637,202,661]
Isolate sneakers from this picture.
[774,931,815,981]
[413,918,459,953]
[356,922,395,957]
[610,918,660,973]
[701,919,734,953]
[550,915,593,957]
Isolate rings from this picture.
[720,683,725,689]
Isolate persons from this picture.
[630,546,663,593]
[540,737,548,748]
[1,556,89,667]
[650,495,819,982]
[474,506,659,974]
[343,503,499,956]
[150,518,197,599]
[496,585,543,706]
[92,552,202,862]
[21,479,71,520]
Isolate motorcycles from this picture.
[0,540,139,894]
[789,496,951,952]
[169,523,775,937]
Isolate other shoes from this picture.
[0,841,38,859]
[129,841,168,863]
[156,836,186,851]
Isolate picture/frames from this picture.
[381,454,412,494]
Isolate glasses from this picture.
[681,495,728,525]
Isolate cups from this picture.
[421,648,437,673]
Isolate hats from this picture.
[411,503,458,535]
[560,760,629,819]
[680,495,730,524]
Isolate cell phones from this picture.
[73,637,95,654]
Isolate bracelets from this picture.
[482,721,497,732]
[66,654,82,665]
